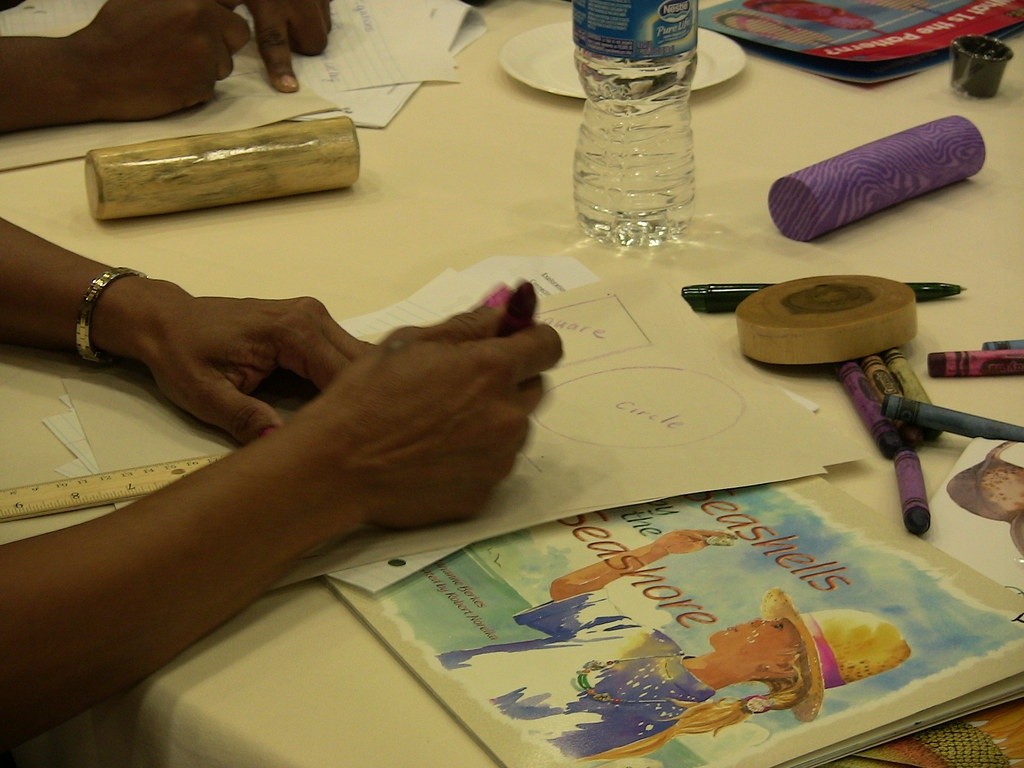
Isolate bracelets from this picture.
[75,266,149,366]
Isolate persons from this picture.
[0,1,336,136]
[0,203,566,755]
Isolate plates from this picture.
[499,22,746,99]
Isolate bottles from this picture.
[573,0,697,246]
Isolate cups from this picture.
[950,36,1014,98]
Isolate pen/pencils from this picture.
[681,280,968,314]
